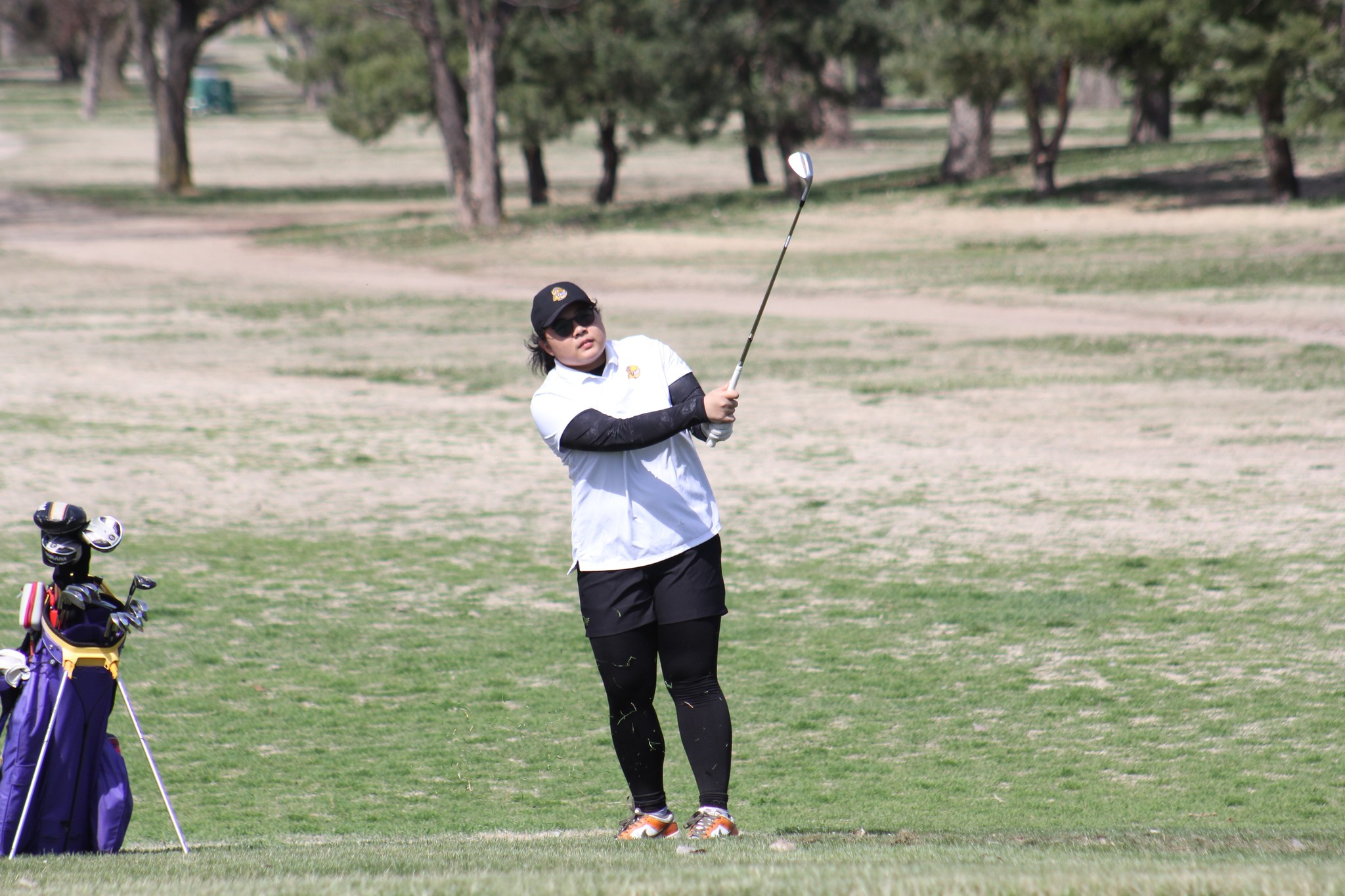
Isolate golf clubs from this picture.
[32,501,159,639]
[704,150,814,449]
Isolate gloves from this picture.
[702,421,734,441]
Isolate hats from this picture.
[531,282,596,331]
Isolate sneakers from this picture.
[613,797,683,839]
[683,807,740,838]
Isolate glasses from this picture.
[552,308,595,337]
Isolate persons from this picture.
[527,280,742,840]
